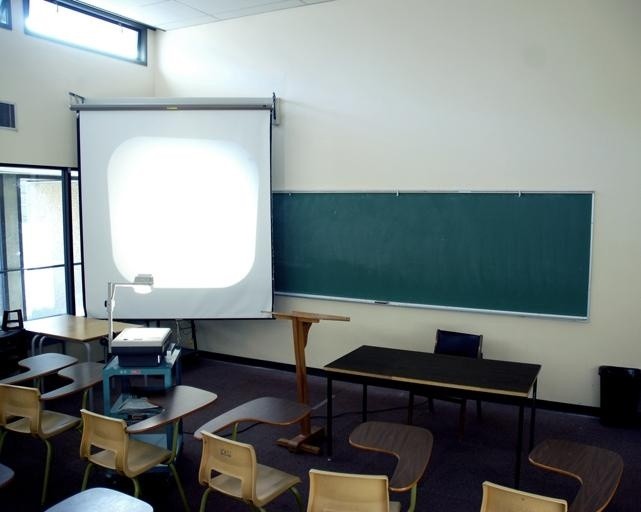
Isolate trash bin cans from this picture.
[599,366,641,429]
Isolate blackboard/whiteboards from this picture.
[274,190,595,321]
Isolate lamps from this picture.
[104,273,154,360]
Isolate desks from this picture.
[323,346,542,490]
[7,314,145,413]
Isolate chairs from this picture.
[404,328,484,432]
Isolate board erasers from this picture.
[375,301,388,304]
